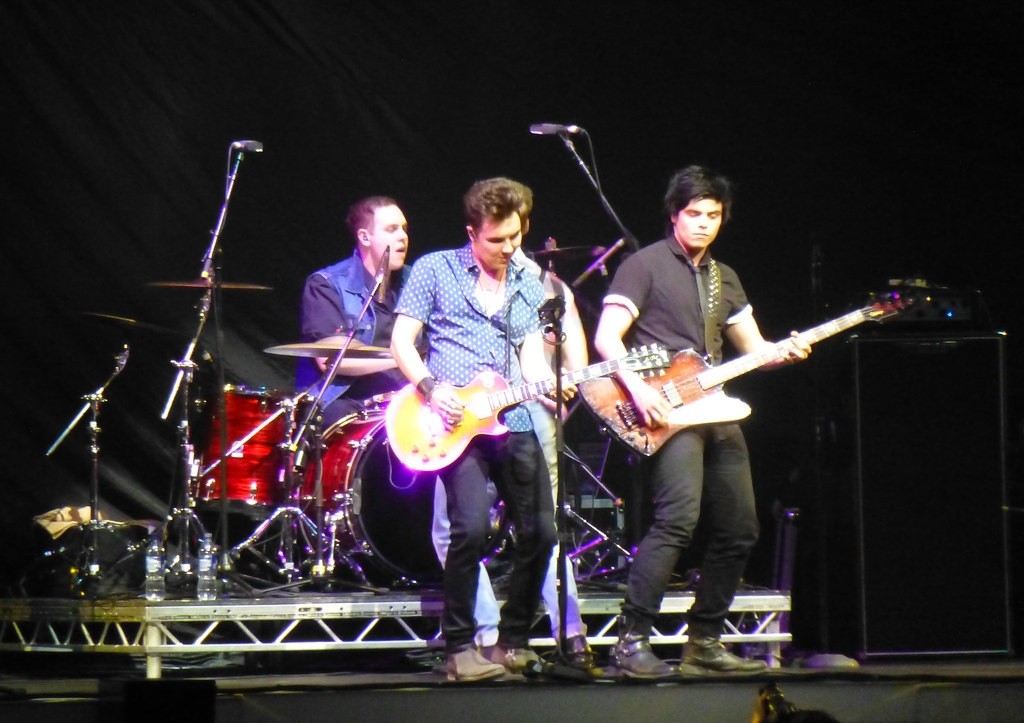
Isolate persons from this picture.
[389,176,594,686]
[593,165,811,681]
[294,196,412,428]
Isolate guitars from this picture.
[573,283,919,460]
[386,337,674,476]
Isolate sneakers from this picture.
[490,648,553,682]
[447,648,506,682]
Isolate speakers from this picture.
[819,330,1017,663]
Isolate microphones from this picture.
[374,246,390,303]
[232,141,263,152]
[573,239,626,288]
[530,123,586,134]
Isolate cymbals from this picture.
[74,300,179,340]
[530,243,595,256]
[261,331,391,359]
[148,274,276,291]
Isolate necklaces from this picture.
[478,270,504,313]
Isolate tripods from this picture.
[36,151,389,598]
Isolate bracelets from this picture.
[416,377,439,402]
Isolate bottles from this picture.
[146,531,166,602]
[197,532,218,601]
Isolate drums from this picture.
[198,378,321,512]
[295,406,514,583]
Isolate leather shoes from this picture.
[606,633,680,677]
[679,634,770,675]
